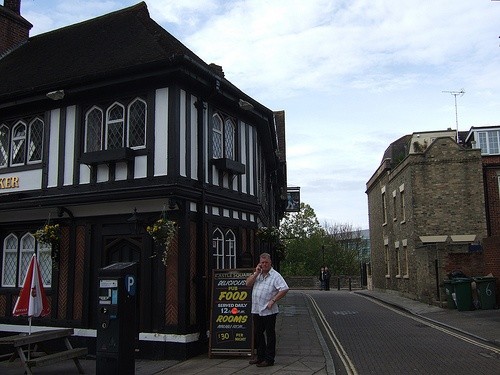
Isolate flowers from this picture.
[147,219,177,267]
[32,225,59,246]
[255,227,282,242]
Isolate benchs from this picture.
[0,350,28,363]
[24,347,87,375]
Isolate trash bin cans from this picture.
[443,279,458,310]
[470,276,497,309]
[456,277,475,311]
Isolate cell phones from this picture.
[258,266,261,270]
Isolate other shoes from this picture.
[257,361,274,367]
[249,358,264,364]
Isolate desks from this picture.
[0,327,84,375]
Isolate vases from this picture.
[152,229,167,241]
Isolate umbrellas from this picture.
[13,253,51,375]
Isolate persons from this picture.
[323,265,331,291]
[246,253,289,368]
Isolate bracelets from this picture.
[254,272,258,276]
[272,298,275,303]
